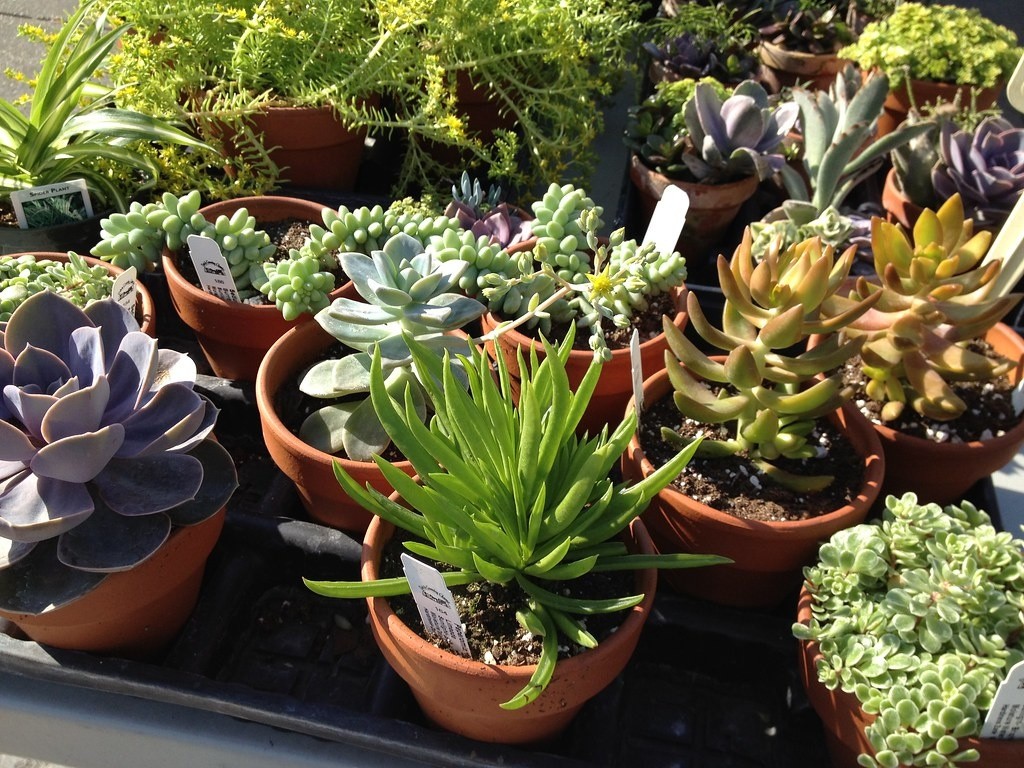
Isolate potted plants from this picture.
[0,0,1022,768]
